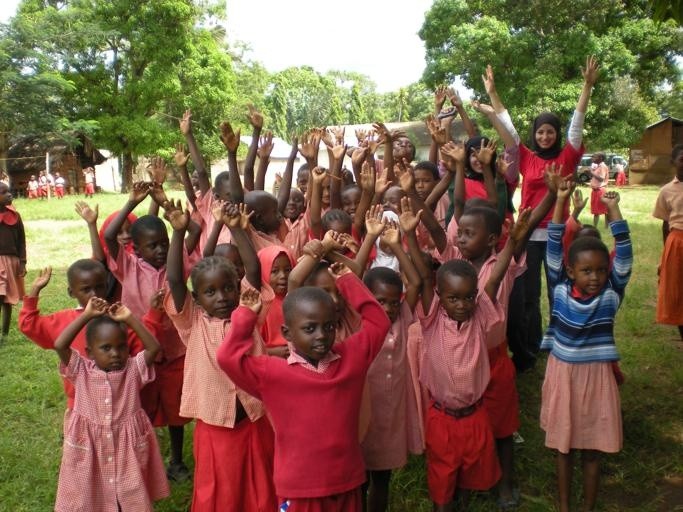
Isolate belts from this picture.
[432,398,483,418]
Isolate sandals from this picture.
[499,494,519,511]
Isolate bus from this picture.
[575,155,629,183]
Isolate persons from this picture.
[0,51,633,512]
[651,143,683,341]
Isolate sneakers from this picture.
[167,460,190,482]
